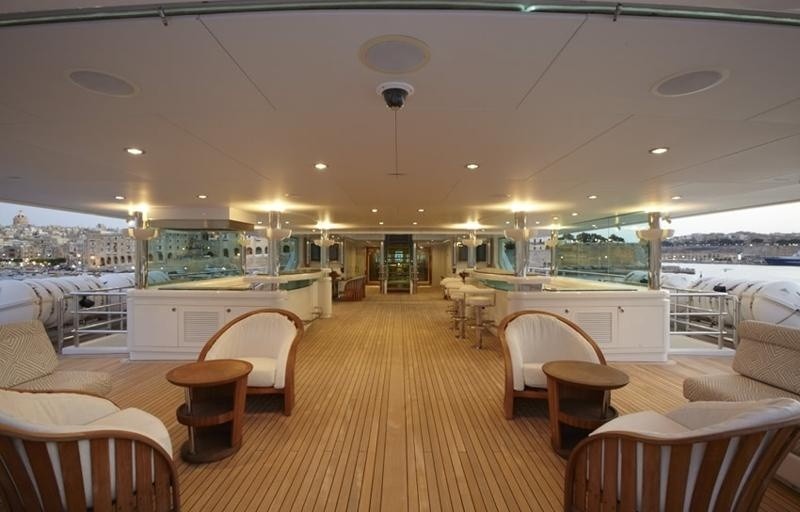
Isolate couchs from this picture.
[496,309,610,420]
[197,307,307,417]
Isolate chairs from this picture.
[0,318,183,510]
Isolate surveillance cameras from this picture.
[380,88,409,112]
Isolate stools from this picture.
[439,276,497,351]
